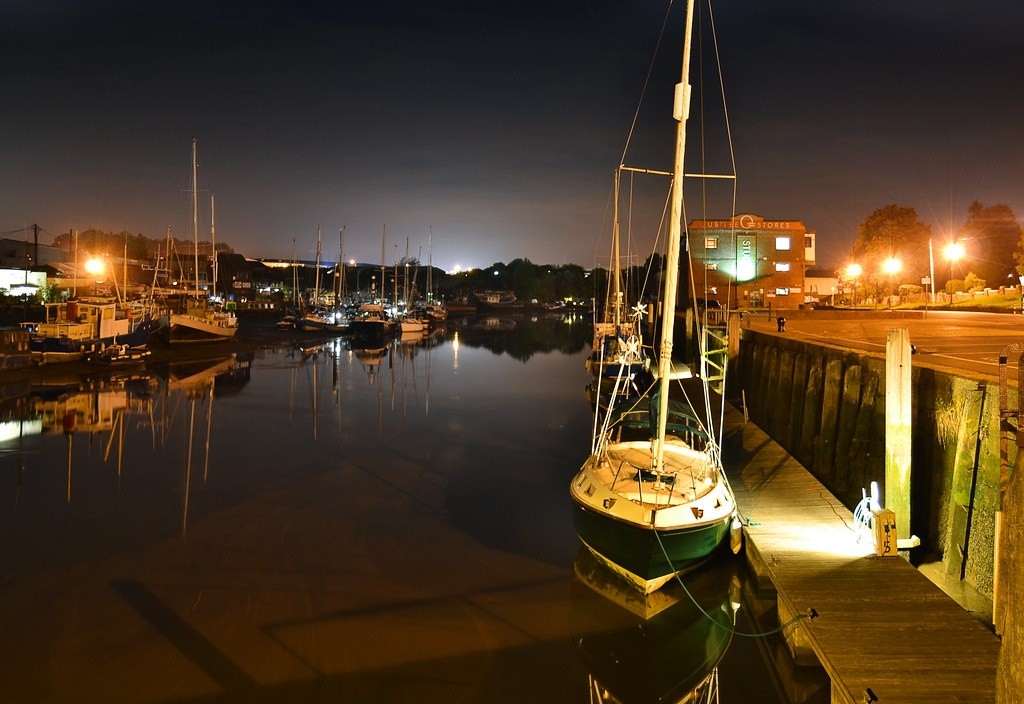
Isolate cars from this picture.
[688,297,723,316]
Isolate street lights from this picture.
[851,267,859,307]
[886,263,896,307]
[947,249,958,304]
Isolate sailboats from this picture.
[289,322,448,440]
[565,1,737,598]
[276,224,448,340]
[590,381,645,444]
[589,168,646,390]
[30,343,238,531]
[567,536,740,704]
[28,138,241,355]
